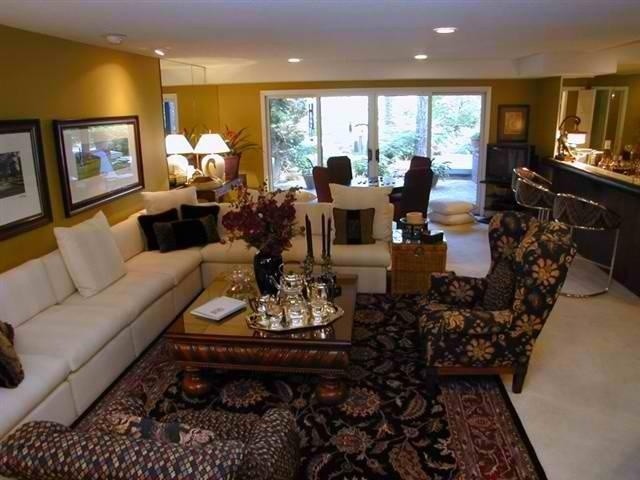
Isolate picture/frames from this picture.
[54,115,145,219]
[0,118,54,240]
[498,104,529,141]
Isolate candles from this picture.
[321,214,326,254]
[305,213,309,257]
[307,219,313,257]
[327,217,331,257]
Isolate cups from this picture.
[266,305,284,326]
[287,298,305,318]
[308,280,329,308]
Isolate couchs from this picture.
[0,203,395,440]
[0,397,297,480]
[418,211,578,393]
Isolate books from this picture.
[189,294,246,321]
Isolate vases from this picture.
[253,248,283,298]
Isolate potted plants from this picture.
[200,123,260,181]
[430,157,453,187]
[296,156,315,189]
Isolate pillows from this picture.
[429,212,479,225]
[430,198,477,215]
[54,211,129,297]
[105,411,215,442]
[138,207,179,250]
[236,184,316,203]
[152,214,221,254]
[141,187,199,220]
[332,207,376,245]
[328,183,394,243]
[181,204,220,226]
[472,257,517,310]
[218,179,305,256]
[0,321,24,389]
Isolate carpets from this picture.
[71,286,549,480]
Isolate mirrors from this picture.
[162,84,219,190]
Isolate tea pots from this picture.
[248,290,280,313]
[265,258,305,297]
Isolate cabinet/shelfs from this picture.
[390,229,448,295]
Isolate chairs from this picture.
[392,154,431,191]
[312,166,334,203]
[510,166,555,221]
[388,169,434,223]
[325,156,353,186]
[552,192,625,301]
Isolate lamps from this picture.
[557,114,588,161]
[165,134,193,186]
[193,134,231,185]
